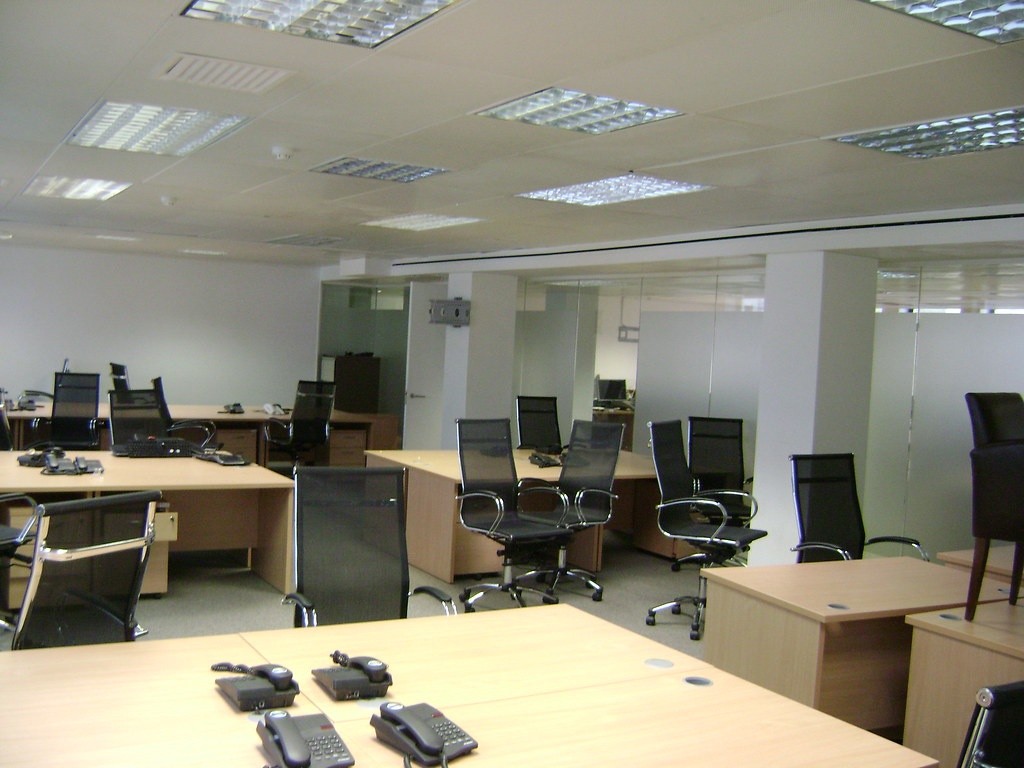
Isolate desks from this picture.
[0,601,942,768]
[902,594,1024,768]
[937,544,1024,587]
[0,450,295,633]
[699,555,1024,732]
[0,403,397,483]
[360,447,708,592]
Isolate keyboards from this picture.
[112,439,194,459]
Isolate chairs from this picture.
[671,416,754,574]
[514,397,564,450]
[0,492,38,631]
[22,371,106,450]
[149,376,225,452]
[0,489,164,650]
[788,452,931,563]
[18,358,71,403]
[263,379,337,473]
[647,419,767,641]
[110,362,149,404]
[963,391,1024,621]
[511,420,626,603]
[281,466,457,628]
[108,389,166,445]
[453,418,574,615]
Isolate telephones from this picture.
[310,647,394,698]
[224,402,244,415]
[263,403,285,416]
[370,702,478,768]
[529,452,560,467]
[17,446,105,475]
[215,664,300,714]
[257,710,356,768]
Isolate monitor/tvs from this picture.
[597,379,626,407]
